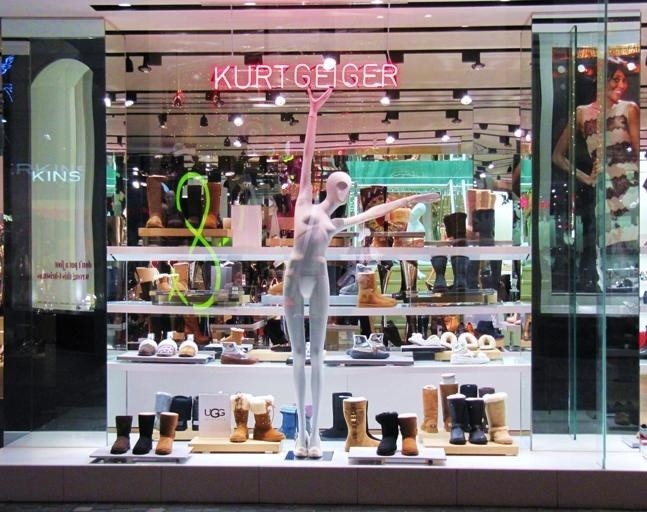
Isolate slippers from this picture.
[346,316,501,365]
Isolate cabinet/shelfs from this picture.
[105,225,532,432]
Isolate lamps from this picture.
[102,48,532,190]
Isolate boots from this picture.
[145,173,221,229]
[230,393,420,457]
[138,315,288,363]
[127,263,244,301]
[109,392,198,455]
[444,212,468,247]
[337,256,521,309]
[420,383,514,446]
[473,209,496,247]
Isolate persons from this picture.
[282,86,439,458]
[553,63,638,292]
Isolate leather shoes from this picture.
[615,409,631,424]
